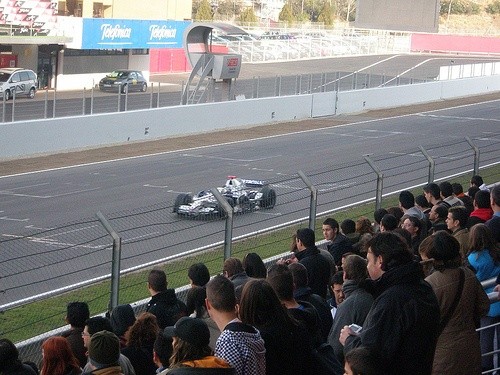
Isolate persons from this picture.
[424,232,491,375]
[204,274,266,375]
[164,316,239,375]
[338,231,439,375]
[80,330,125,375]
[0,175,500,375]
[144,269,187,330]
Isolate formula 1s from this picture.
[172,175,277,220]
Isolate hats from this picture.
[0,338,19,365]
[110,303,136,334]
[88,330,121,367]
[163,316,210,343]
[67,301,91,322]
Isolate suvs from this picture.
[99,69,147,94]
[0,68,39,101]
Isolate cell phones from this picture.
[349,323,363,333]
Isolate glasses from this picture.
[401,223,410,227]
[81,332,91,336]
